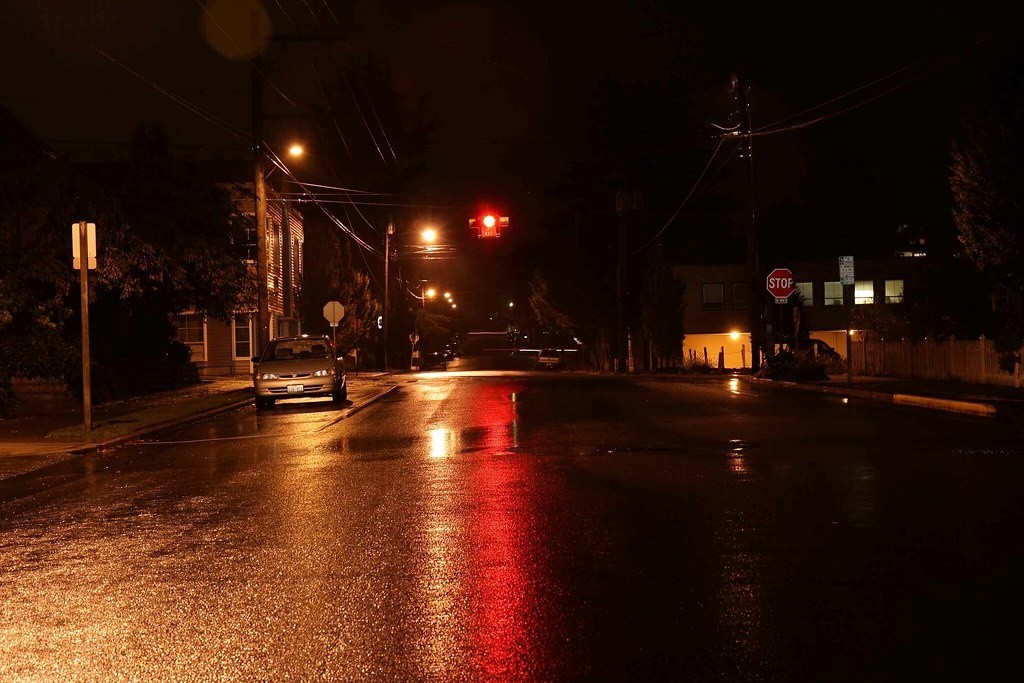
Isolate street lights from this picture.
[421,285,439,309]
[384,221,442,373]
[253,138,310,362]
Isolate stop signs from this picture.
[766,268,798,300]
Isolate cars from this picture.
[808,338,842,369]
[536,349,561,369]
[251,334,349,406]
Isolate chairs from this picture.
[312,345,325,353]
[277,348,294,357]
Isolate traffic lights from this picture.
[481,207,499,237]
